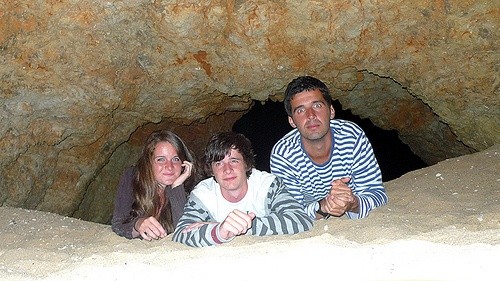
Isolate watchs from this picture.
[314,199,330,219]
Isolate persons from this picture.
[112,129,194,241]
[172,132,314,247]
[270,75,388,219]
